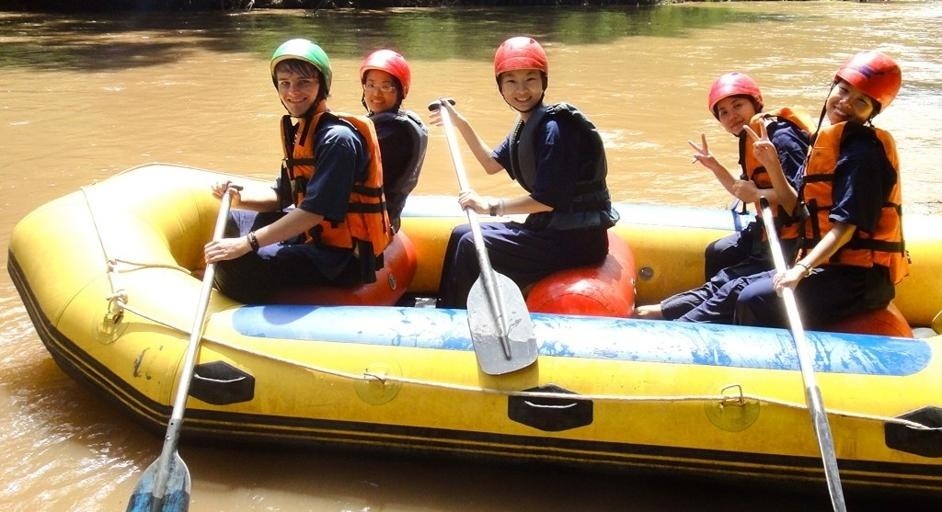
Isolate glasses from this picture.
[363,83,397,92]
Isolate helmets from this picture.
[361,50,410,98]
[270,38,332,94]
[495,37,548,81]
[835,52,901,113]
[709,73,762,119]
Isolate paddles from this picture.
[428,96,540,374]
[126,185,235,512]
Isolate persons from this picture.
[428,36,621,308]
[359,49,428,234]
[634,72,813,319]
[203,37,395,304]
[672,50,909,325]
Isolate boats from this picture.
[6,162,942,502]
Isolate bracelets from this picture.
[797,260,811,280]
[247,231,259,252]
